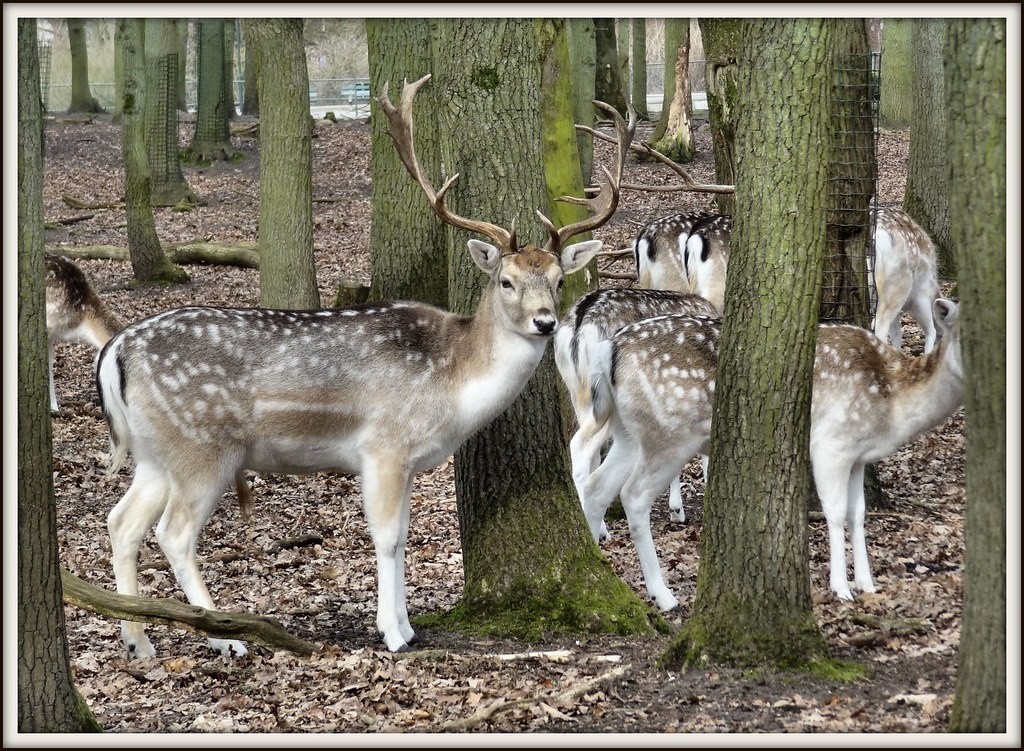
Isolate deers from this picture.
[45,252,120,415]
[98,71,644,659]
[555,125,970,611]
